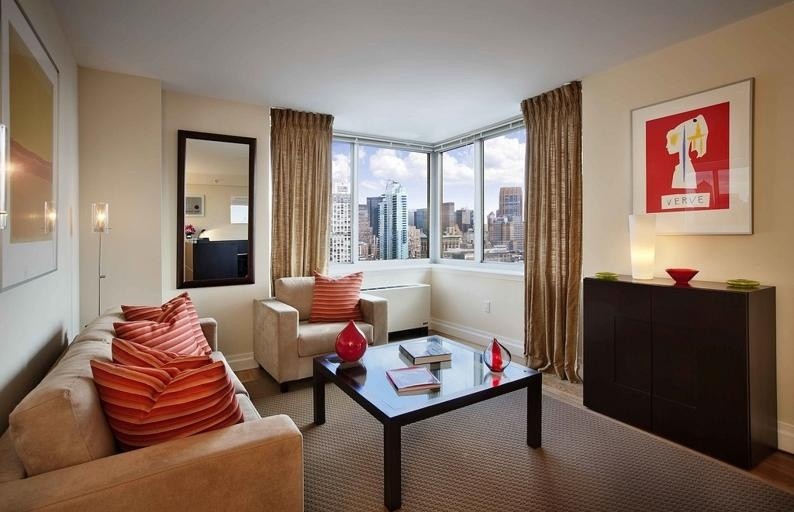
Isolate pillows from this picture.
[83,358,247,450]
[120,289,211,354]
[111,337,214,371]
[112,295,211,362]
[307,267,367,324]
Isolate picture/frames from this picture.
[625,75,756,238]
[0,1,64,297]
[185,193,205,217]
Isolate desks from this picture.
[191,239,249,281]
[306,334,549,512]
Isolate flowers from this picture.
[184,224,195,235]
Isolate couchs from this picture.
[252,273,391,395]
[0,303,306,511]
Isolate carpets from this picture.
[236,376,794,512]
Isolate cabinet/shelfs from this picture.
[577,274,786,474]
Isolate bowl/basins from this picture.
[666,266,699,284]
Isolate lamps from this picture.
[198,226,211,243]
[89,201,113,319]
[623,212,659,283]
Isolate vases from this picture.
[185,234,192,240]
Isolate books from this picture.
[386,366,440,391]
[399,339,452,365]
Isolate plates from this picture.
[595,271,617,279]
[728,279,760,289]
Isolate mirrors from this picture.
[177,127,256,290]
